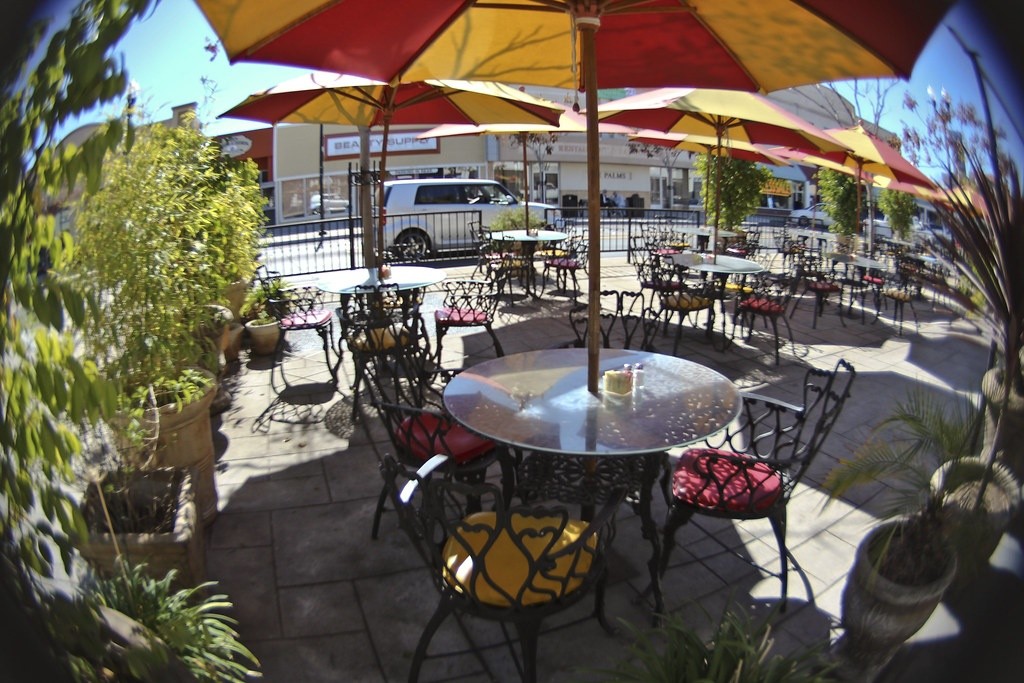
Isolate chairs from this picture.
[257,206,957,683]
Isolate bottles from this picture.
[531,228,538,236]
[382,263,387,278]
[632,362,645,388]
[623,365,633,380]
[386,262,391,277]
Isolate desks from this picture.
[905,252,942,304]
[676,225,739,277]
[483,226,569,286]
[669,253,765,330]
[819,249,886,318]
[438,346,744,590]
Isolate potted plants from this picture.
[0,0,283,683]
[805,380,1008,683]
[896,92,1024,570]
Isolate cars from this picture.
[310,194,350,215]
[788,202,836,227]
[862,213,932,241]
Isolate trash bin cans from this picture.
[562,194,578,217]
[627,193,645,219]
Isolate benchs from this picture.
[316,263,450,378]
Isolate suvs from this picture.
[371,179,565,261]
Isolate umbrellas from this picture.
[192,0,962,392]
